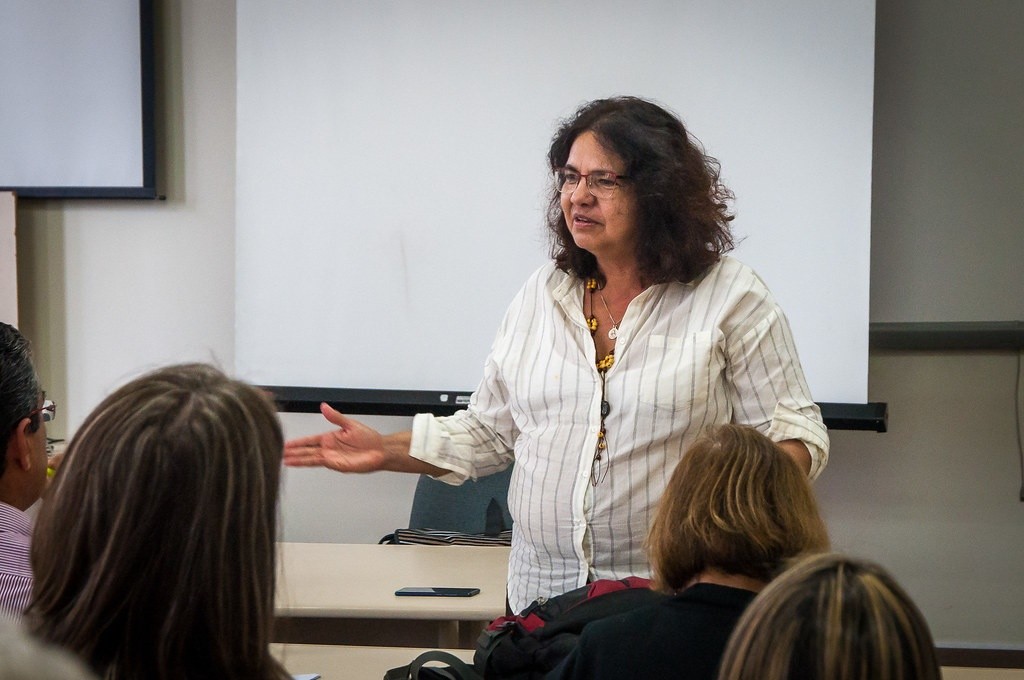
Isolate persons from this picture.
[0,320,53,629]
[275,93,830,621]
[1,362,295,680]
[571,421,944,680]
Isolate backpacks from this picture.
[384,576,675,680]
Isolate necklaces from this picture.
[586,271,668,488]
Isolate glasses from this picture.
[553,167,635,199]
[15,398,57,431]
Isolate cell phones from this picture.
[395,586,481,598]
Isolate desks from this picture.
[266,641,478,680]
[271,538,511,650]
